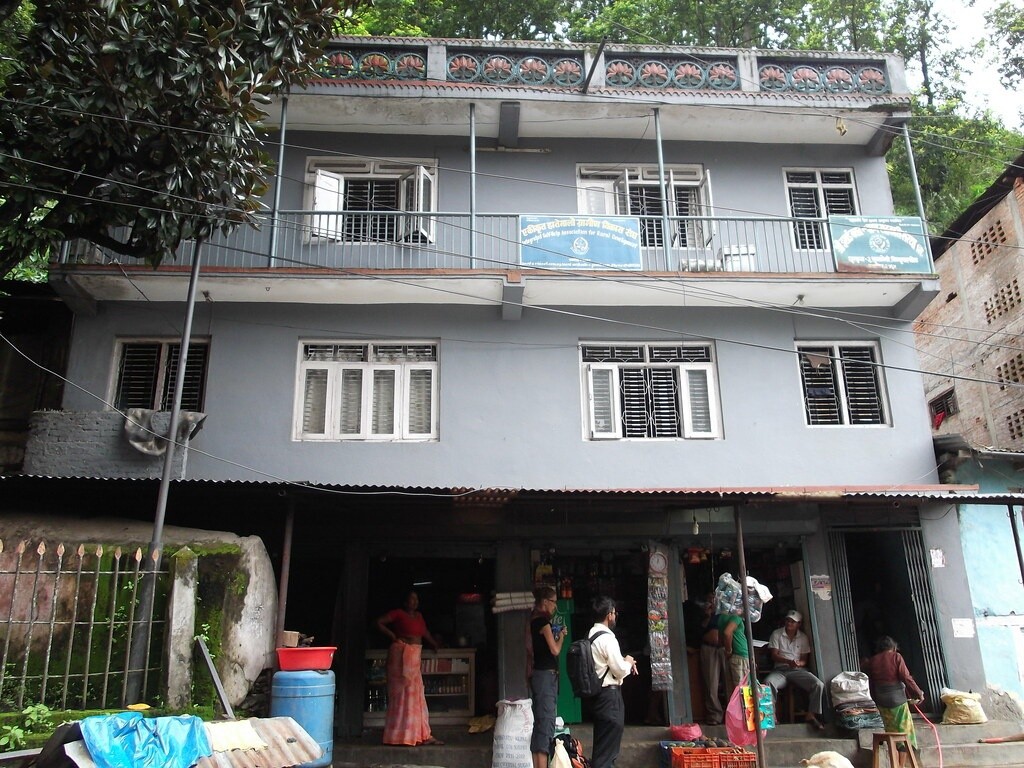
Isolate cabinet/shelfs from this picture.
[686,645,768,723]
[364,647,476,727]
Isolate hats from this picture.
[785,610,802,622]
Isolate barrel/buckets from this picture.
[269,670,335,768]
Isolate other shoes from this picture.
[706,718,717,726]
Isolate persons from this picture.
[860,634,925,768]
[529,586,568,768]
[699,576,825,731]
[566,595,639,768]
[377,590,446,747]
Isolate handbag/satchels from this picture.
[725,671,775,746]
[550,738,573,768]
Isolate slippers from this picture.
[422,737,445,745]
[807,717,825,730]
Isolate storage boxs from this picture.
[657,736,757,768]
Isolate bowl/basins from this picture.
[276,647,337,671]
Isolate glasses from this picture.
[546,598,558,604]
[608,611,618,617]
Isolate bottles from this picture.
[368,689,372,713]
[567,581,572,598]
[425,681,462,694]
[372,660,386,676]
[374,690,379,711]
[561,581,566,598]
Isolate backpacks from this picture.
[566,630,612,697]
[682,599,715,649]
[549,733,591,768]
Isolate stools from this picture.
[872,732,918,768]
[781,684,807,723]
